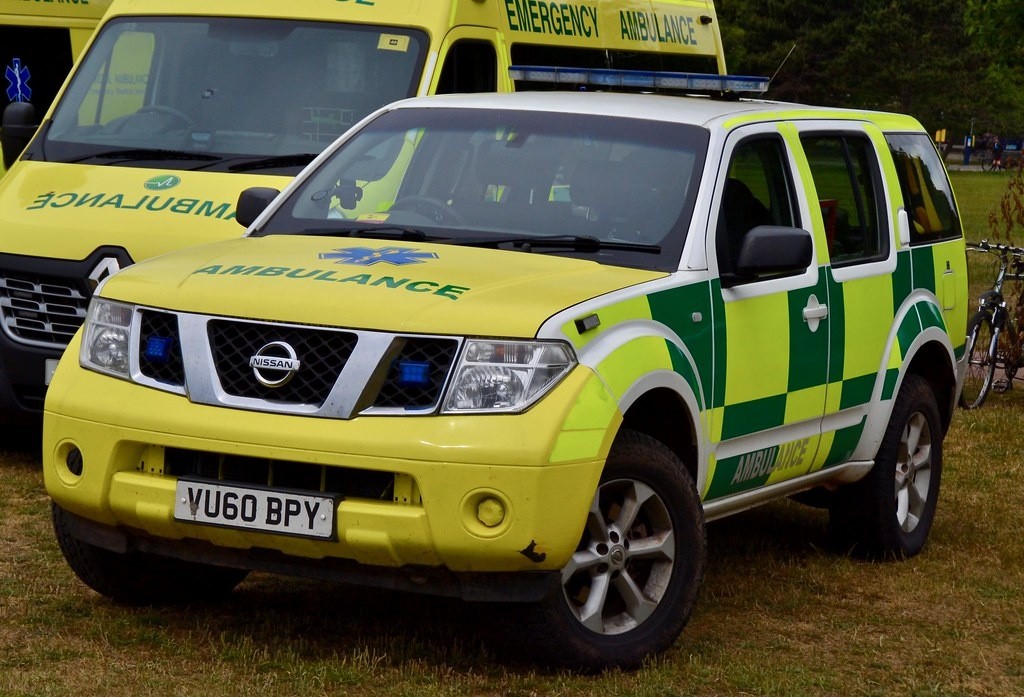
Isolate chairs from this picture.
[452,140,536,185]
[647,186,723,253]
[171,46,279,133]
[571,161,630,207]
[722,179,775,251]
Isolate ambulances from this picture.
[0,0,158,176]
[45,68,975,667]
[0,1,728,446]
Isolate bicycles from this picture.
[980,138,1016,173]
[963,240,1023,407]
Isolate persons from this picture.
[991,135,1003,171]
